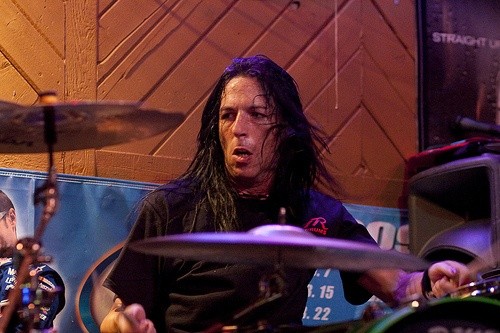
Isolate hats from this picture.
[0,190,14,222]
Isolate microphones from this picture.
[447,114,500,138]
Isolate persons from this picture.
[0,189,67,333]
[97,54,475,331]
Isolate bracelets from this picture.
[421,267,438,301]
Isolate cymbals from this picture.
[128,223,432,274]
[0,100,185,153]
